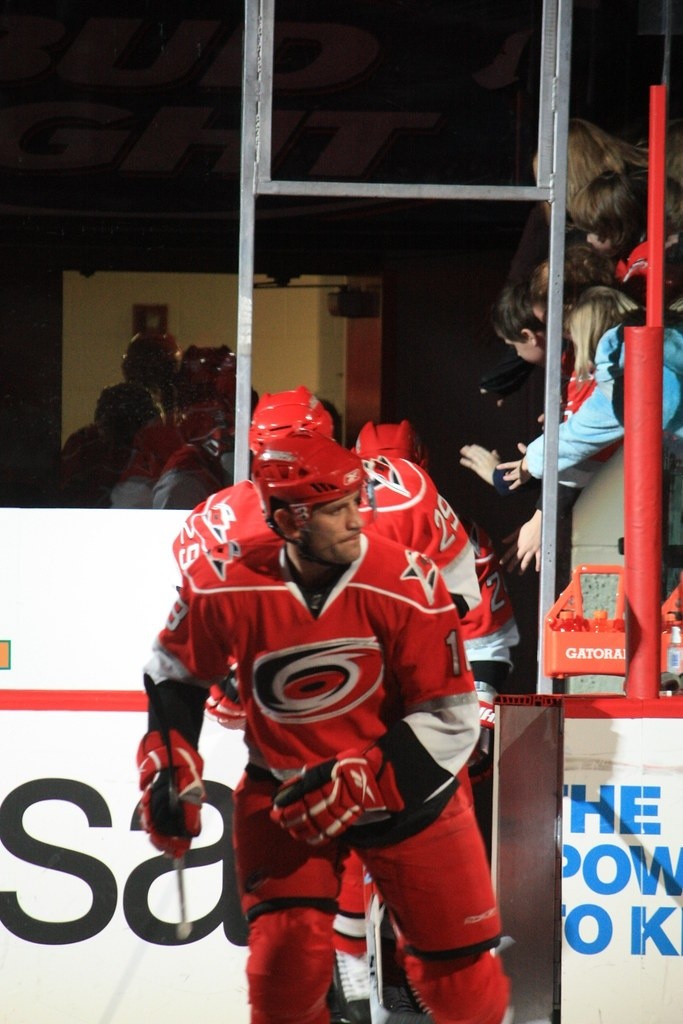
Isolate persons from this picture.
[141,385,518,1024]
[60,332,260,507]
[459,118,683,661]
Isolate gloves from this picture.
[136,729,205,859]
[271,743,405,849]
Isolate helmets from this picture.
[176,389,234,452]
[122,329,178,385]
[251,427,368,529]
[179,342,235,399]
[248,384,333,456]
[351,420,431,476]
[95,382,162,447]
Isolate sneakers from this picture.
[331,948,373,1024]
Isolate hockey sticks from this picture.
[140,672,180,818]
